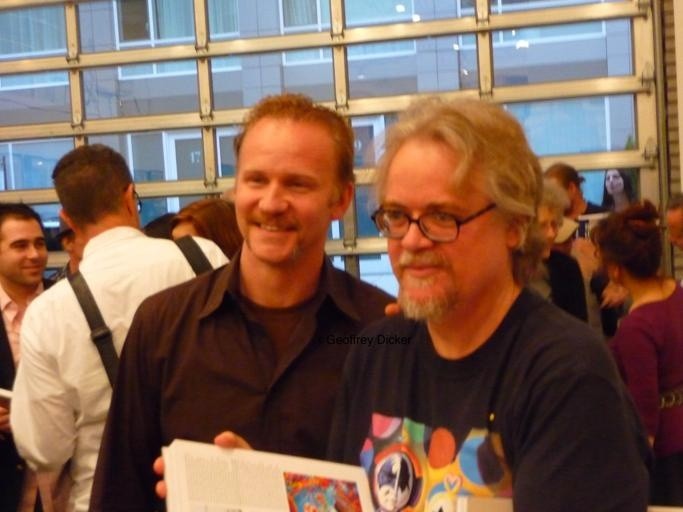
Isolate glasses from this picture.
[369,202,494,241]
[123,187,142,212]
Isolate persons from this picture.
[151,92,651,512]
[88,95,401,512]
[1,143,242,512]
[539,161,682,511]
